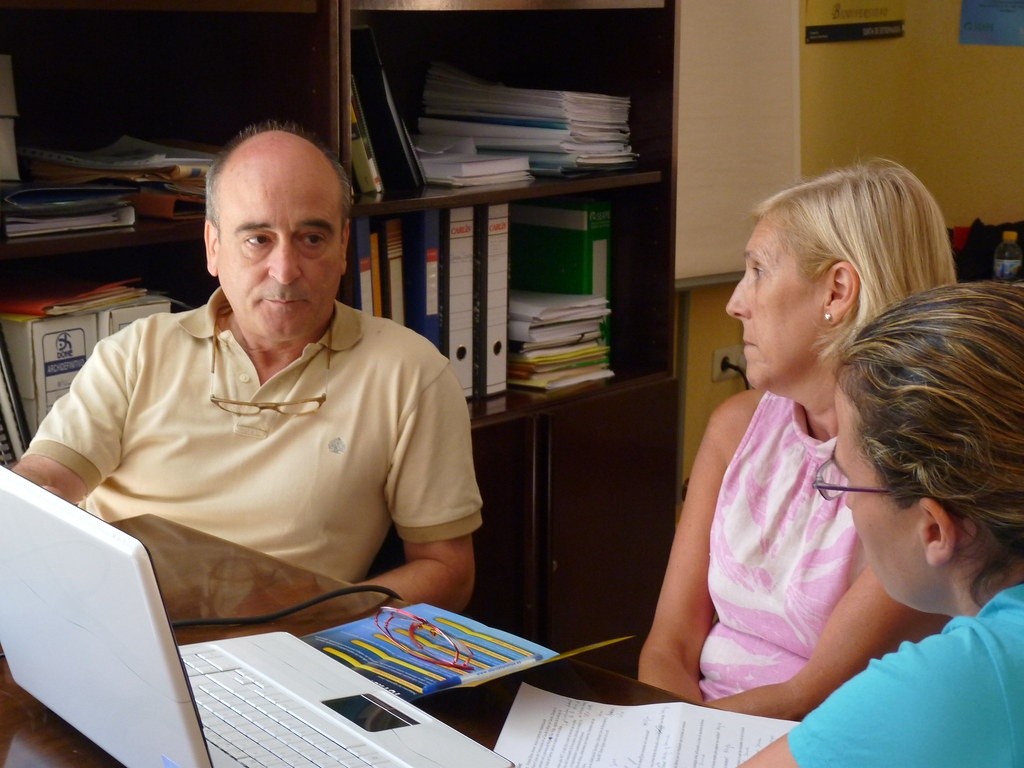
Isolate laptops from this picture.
[1,467,515,768]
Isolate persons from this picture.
[737,284,1023,767]
[640,159,958,724]
[12,124,485,610]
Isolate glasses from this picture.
[208,305,334,417]
[373,604,477,674]
[810,458,893,502]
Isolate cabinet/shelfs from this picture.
[1,0,680,676]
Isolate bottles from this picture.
[989,229,1023,283]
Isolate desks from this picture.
[0,513,694,768]
